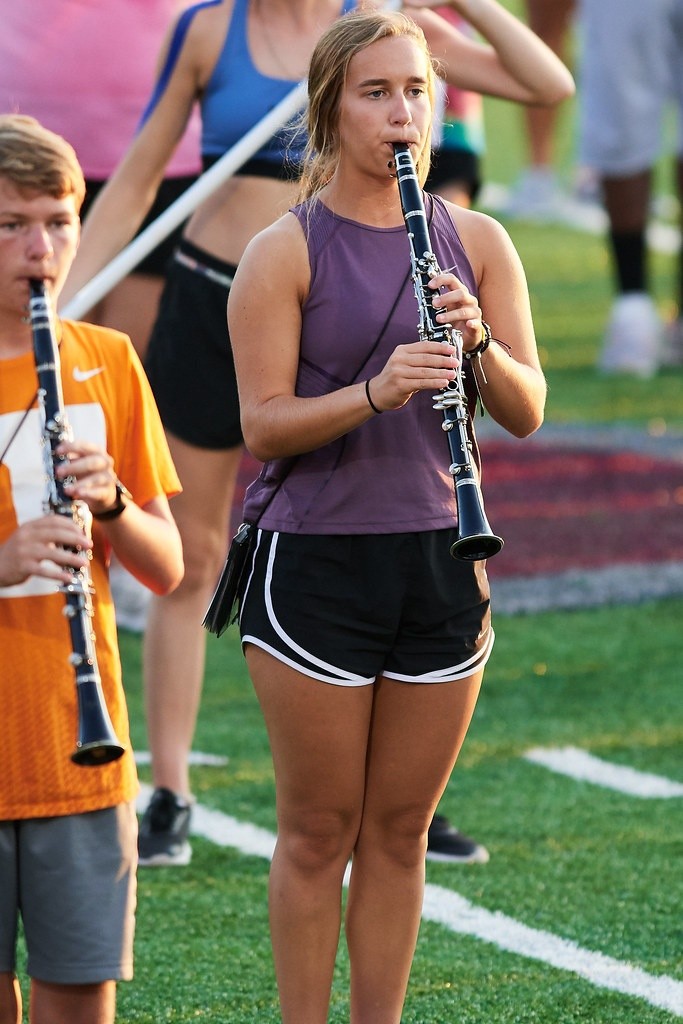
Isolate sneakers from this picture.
[659,313,683,371]
[427,814,490,865]
[592,288,658,378]
[135,786,196,869]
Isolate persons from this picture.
[65,0,497,866]
[1,108,192,1018]
[220,16,553,1023]
[1,0,682,626]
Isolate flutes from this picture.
[28,278,128,766]
[391,141,507,560]
